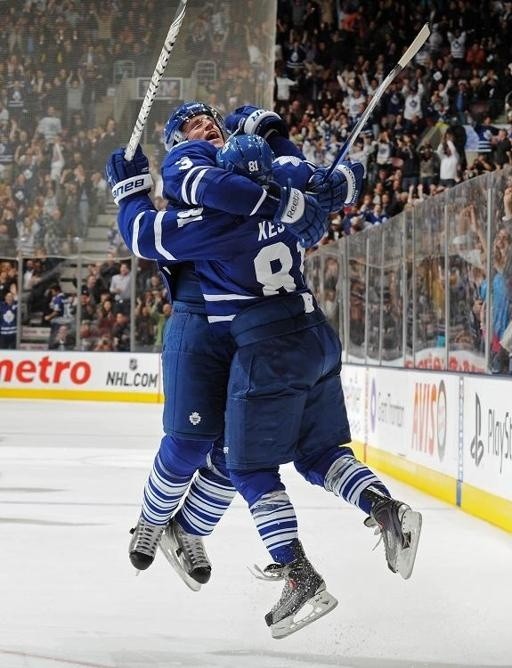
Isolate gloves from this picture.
[225,105,289,140]
[105,143,153,206]
[267,179,331,247]
[308,160,364,215]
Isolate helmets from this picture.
[163,101,225,152]
[215,134,273,178]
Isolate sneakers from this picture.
[247,538,326,625]
[361,484,412,573]
[165,515,211,583]
[129,514,167,569]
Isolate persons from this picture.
[125,101,364,584]
[0,1,512,375]
[105,105,414,629]
